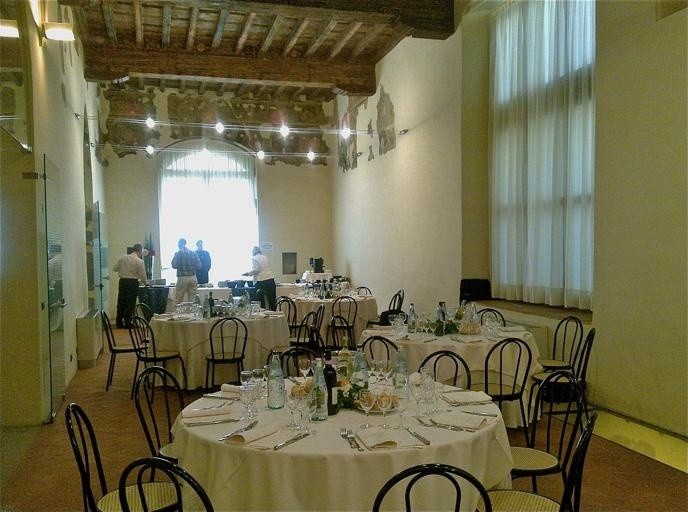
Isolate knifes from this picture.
[441,388,470,395]
[459,408,498,417]
[202,393,241,402]
[275,433,309,449]
[406,428,431,446]
[185,419,238,429]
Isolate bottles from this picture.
[195,291,201,313]
[208,292,215,316]
[305,277,342,299]
[267,349,286,409]
[354,343,368,391]
[310,356,328,420]
[337,335,354,377]
[408,302,418,332]
[393,344,407,388]
[202,294,209,318]
[323,348,341,416]
[455,300,466,321]
[228,293,235,311]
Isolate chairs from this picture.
[397,288,405,308]
[101,310,147,392]
[205,316,248,390]
[366,294,402,330]
[472,337,533,448]
[477,412,598,511]
[133,304,153,344]
[267,312,317,370]
[278,300,297,337]
[290,302,323,344]
[118,457,214,512]
[363,337,398,368]
[326,296,358,347]
[332,316,352,350]
[417,350,472,390]
[127,317,187,400]
[64,404,178,512]
[510,371,581,494]
[538,316,583,373]
[280,348,318,378]
[529,328,596,448]
[271,296,309,338]
[372,464,492,512]
[134,366,184,481]
[357,287,371,295]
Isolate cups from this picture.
[175,302,193,319]
[195,306,203,320]
[485,321,500,340]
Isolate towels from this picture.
[353,424,399,450]
[222,411,281,445]
[179,405,232,417]
[220,382,242,393]
[432,409,487,433]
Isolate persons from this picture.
[171,239,201,307]
[193,240,211,284]
[243,247,277,311]
[113,244,149,330]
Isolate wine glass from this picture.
[358,389,376,431]
[298,395,316,435]
[397,313,407,331]
[263,365,270,387]
[227,306,234,317]
[422,319,431,340]
[374,390,394,432]
[366,360,374,378]
[416,318,427,339]
[428,317,438,340]
[299,358,313,384]
[281,391,301,432]
[388,315,396,334]
[248,369,268,398]
[371,359,384,382]
[214,304,221,320]
[240,371,253,392]
[313,287,321,301]
[380,360,393,386]
[432,384,447,412]
[239,388,256,425]
[392,391,410,431]
[423,383,438,416]
[219,306,227,319]
[321,284,329,302]
[419,367,433,388]
[408,383,426,419]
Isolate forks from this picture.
[446,401,486,409]
[192,401,226,411]
[450,399,494,404]
[347,430,363,450]
[341,428,354,449]
[286,375,304,387]
[416,418,465,432]
[219,420,258,443]
[355,430,373,451]
[423,337,441,343]
[429,420,473,433]
[195,403,233,412]
